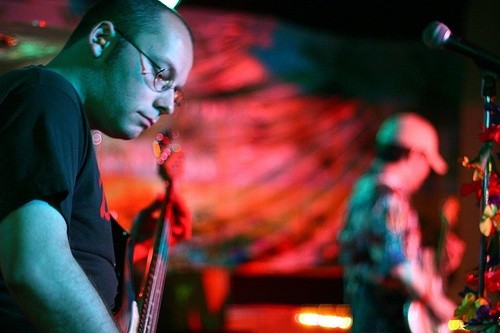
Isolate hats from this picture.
[376,112,448,175]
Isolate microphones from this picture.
[422,21,500,74]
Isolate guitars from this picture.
[128,126,183,333]
[403,214,457,333]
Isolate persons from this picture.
[93,58,94,61]
[334,114,466,333]
[0,0,194,333]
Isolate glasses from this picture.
[115,28,184,106]
